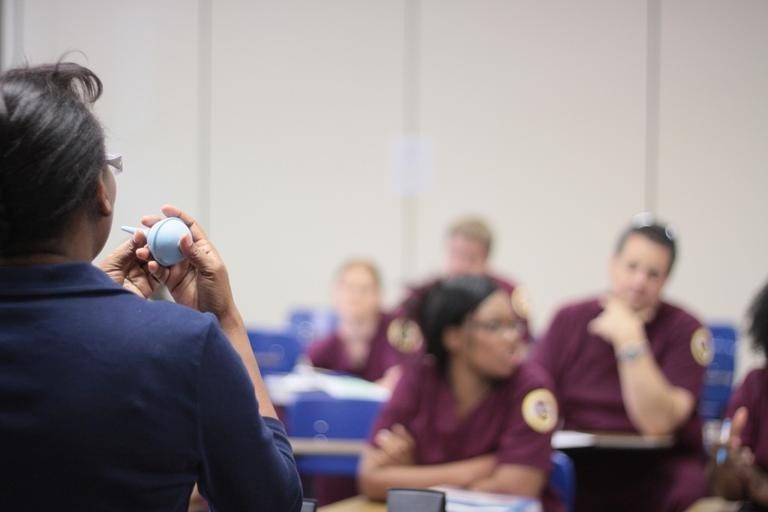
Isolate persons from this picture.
[685,277,768,512]
[304,257,426,505]
[354,274,567,512]
[388,211,530,347]
[0,50,304,512]
[543,214,712,512]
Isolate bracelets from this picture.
[615,341,652,363]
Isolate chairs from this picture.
[236,309,765,512]
